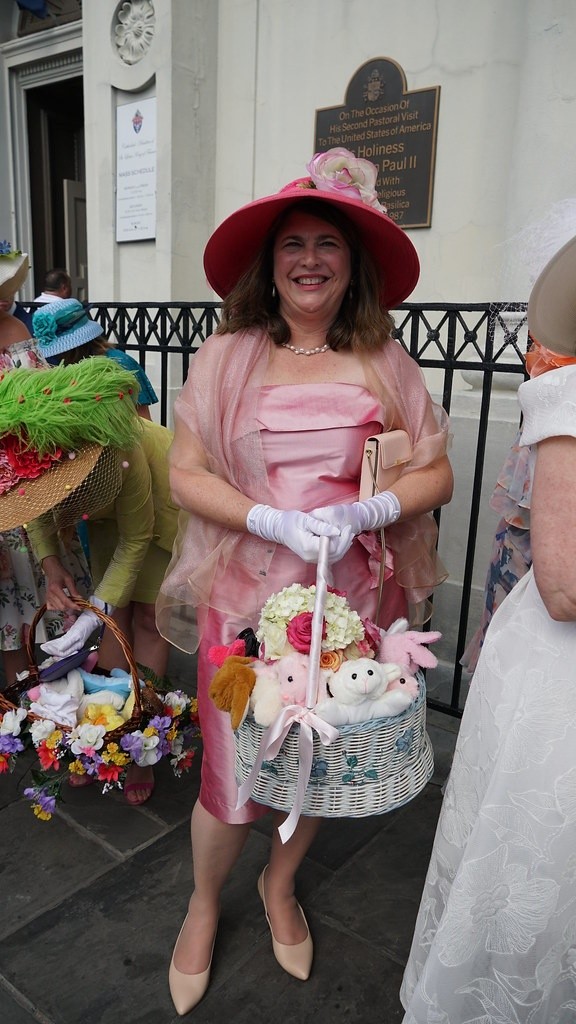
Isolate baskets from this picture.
[0,596,162,766]
[228,535,436,817]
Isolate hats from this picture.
[203,147,420,313]
[527,235,576,359]
[31,297,105,358]
[0,240,30,301]
[0,356,141,533]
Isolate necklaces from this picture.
[282,342,330,355]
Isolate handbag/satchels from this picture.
[359,429,412,502]
[40,603,108,681]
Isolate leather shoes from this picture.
[168,903,221,1015]
[257,864,312,981]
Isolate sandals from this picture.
[68,773,94,787]
[124,782,154,805]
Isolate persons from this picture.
[0,358,188,684]
[399,235,575,1023]
[0,244,159,685]
[157,147,454,1015]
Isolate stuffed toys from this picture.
[28,668,147,730]
[207,617,444,730]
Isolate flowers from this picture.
[256,582,383,673]
[306,146,388,213]
[0,688,203,820]
[0,239,21,260]
[0,428,63,495]
[31,311,58,349]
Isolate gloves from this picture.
[307,490,401,535]
[40,595,117,657]
[247,504,356,566]
